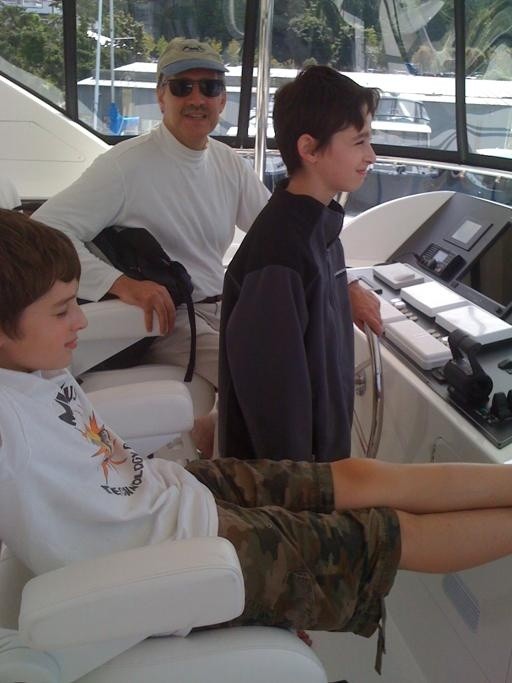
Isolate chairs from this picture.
[12,299,215,460]
[0,535,328,683]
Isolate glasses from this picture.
[157,78,225,97]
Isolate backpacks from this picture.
[77,225,197,382]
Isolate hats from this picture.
[157,37,228,76]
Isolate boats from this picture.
[81,57,512,159]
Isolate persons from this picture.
[218,61,384,460]
[30,36,384,466]
[1,207,511,637]
